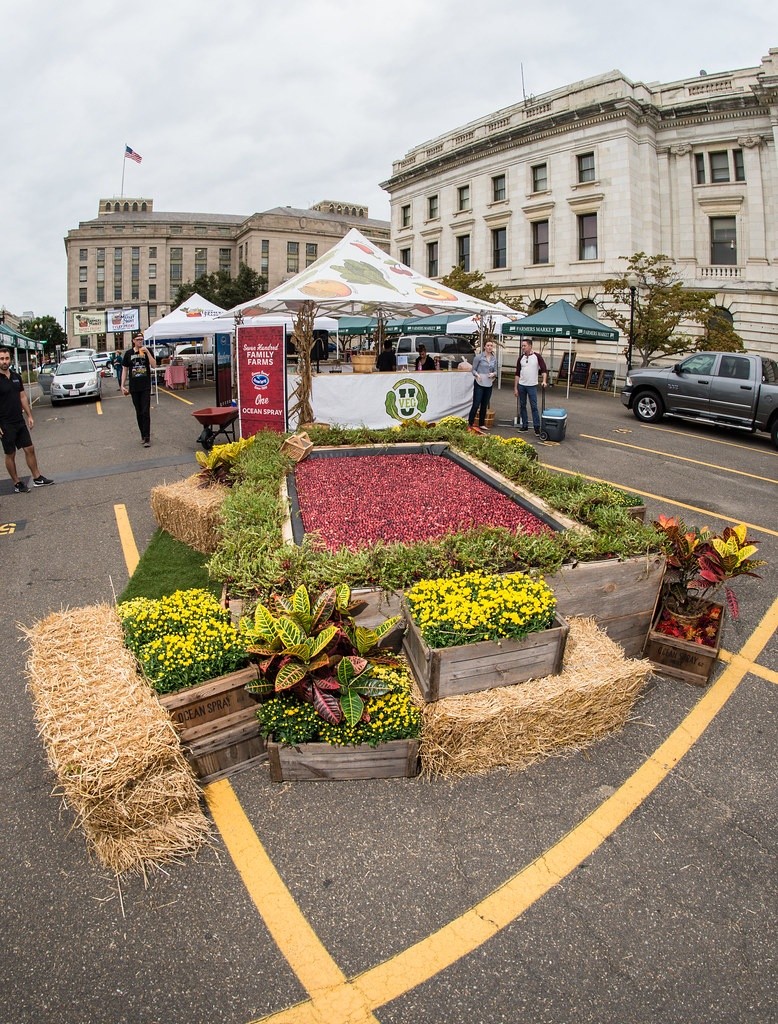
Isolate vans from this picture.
[395,333,476,372]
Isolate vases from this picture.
[400,607,570,703]
[158,662,267,784]
[267,735,419,782]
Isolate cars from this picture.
[328,342,337,352]
[38,359,104,407]
[63,347,115,377]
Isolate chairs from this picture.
[188,363,202,381]
[168,366,185,391]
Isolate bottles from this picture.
[437,360,440,370]
[418,360,422,371]
[448,360,452,371]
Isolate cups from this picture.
[138,347,145,356]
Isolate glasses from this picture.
[133,338,144,341]
[526,356,528,363]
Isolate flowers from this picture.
[404,569,558,648]
[115,588,252,695]
[255,652,422,747]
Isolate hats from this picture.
[133,331,143,339]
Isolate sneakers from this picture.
[33,475,54,487]
[518,427,528,432]
[14,482,31,493]
[534,429,540,436]
[480,425,488,430]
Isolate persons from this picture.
[0,348,55,493]
[468,340,498,430]
[415,344,436,371]
[29,350,54,369]
[376,340,397,373]
[114,350,123,390]
[121,332,156,447]
[514,339,547,436]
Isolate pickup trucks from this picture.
[144,345,172,383]
[169,344,230,370]
[624,354,778,452]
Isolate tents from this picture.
[143,292,338,349]
[501,299,619,397]
[0,323,47,407]
[329,311,475,336]
[214,228,528,370]
[446,301,528,389]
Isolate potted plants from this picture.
[637,512,767,686]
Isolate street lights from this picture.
[621,286,637,394]
[33,324,43,381]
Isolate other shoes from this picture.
[144,442,151,447]
[141,440,145,443]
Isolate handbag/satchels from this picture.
[457,355,473,371]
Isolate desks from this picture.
[152,367,188,391]
[286,354,475,430]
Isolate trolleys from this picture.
[191,407,239,450]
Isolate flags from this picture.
[124,146,141,163]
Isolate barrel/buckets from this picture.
[473,409,496,428]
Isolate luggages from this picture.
[540,385,568,441]
